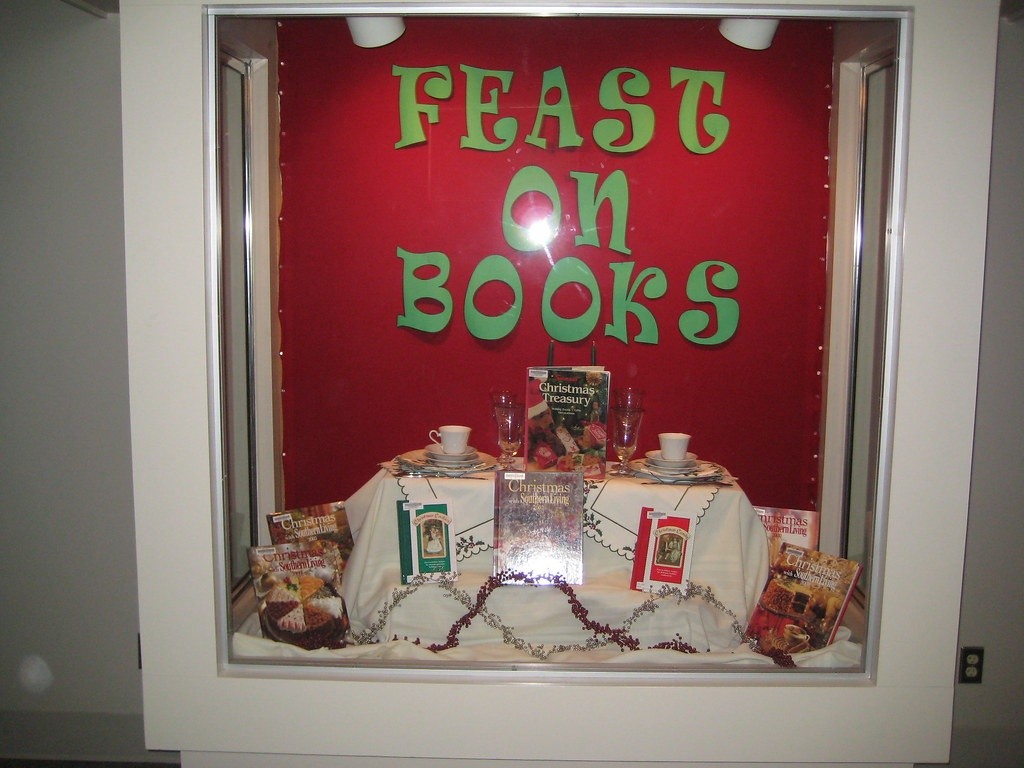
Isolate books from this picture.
[525,364,609,480]
[754,505,819,563]
[395,499,459,585]
[246,540,354,651]
[738,541,862,654]
[492,472,583,587]
[630,507,697,596]
[266,500,354,583]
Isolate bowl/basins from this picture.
[425,443,477,460]
[645,450,699,468]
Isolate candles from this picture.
[548,340,554,366]
[591,341,596,366]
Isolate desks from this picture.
[332,455,772,652]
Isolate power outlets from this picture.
[959,646,985,684]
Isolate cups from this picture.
[659,432,691,460]
[785,625,811,646]
[429,425,472,454]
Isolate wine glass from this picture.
[492,392,524,472]
[608,387,646,479]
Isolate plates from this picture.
[400,449,497,475]
[643,460,702,474]
[420,452,480,467]
[628,458,720,482]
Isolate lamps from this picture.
[719,17,781,50]
[343,15,406,49]
